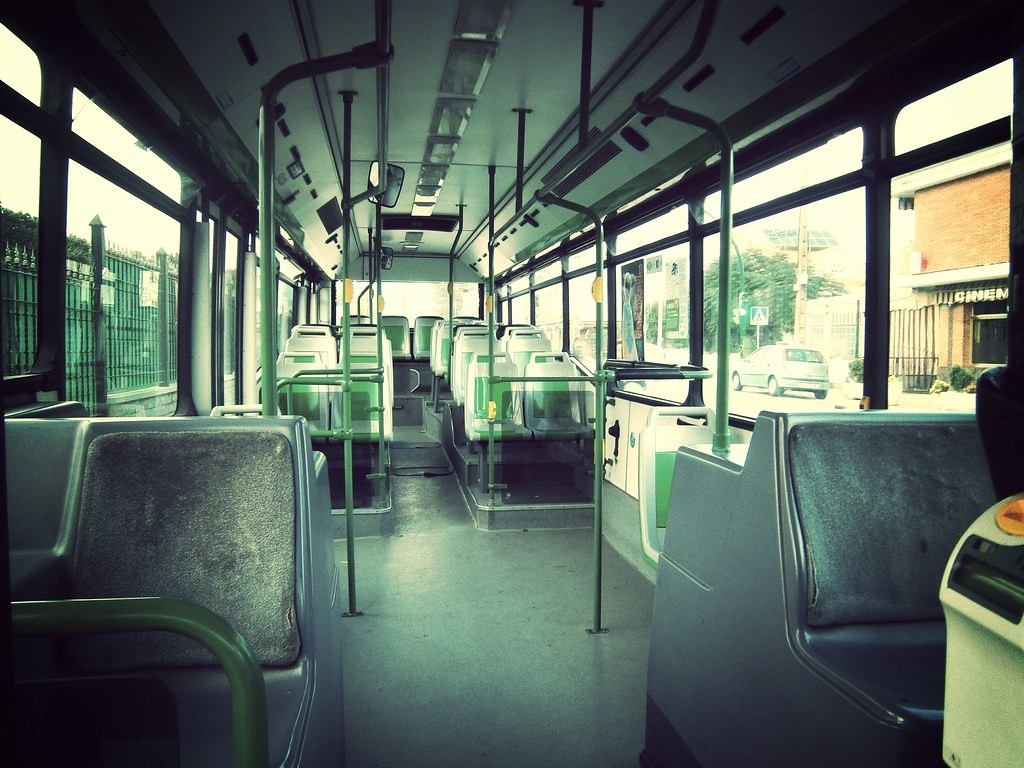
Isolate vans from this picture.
[572,335,597,359]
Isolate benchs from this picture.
[777,416,996,728]
[0,416,315,768]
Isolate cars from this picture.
[729,344,830,399]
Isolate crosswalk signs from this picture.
[750,306,768,326]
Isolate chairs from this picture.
[523,352,595,442]
[505,329,555,404]
[276,351,330,445]
[638,407,731,570]
[340,315,505,414]
[329,351,394,496]
[284,326,338,404]
[464,352,532,494]
[451,326,504,455]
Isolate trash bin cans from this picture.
[938,493,1024,768]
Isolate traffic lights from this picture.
[738,293,748,316]
